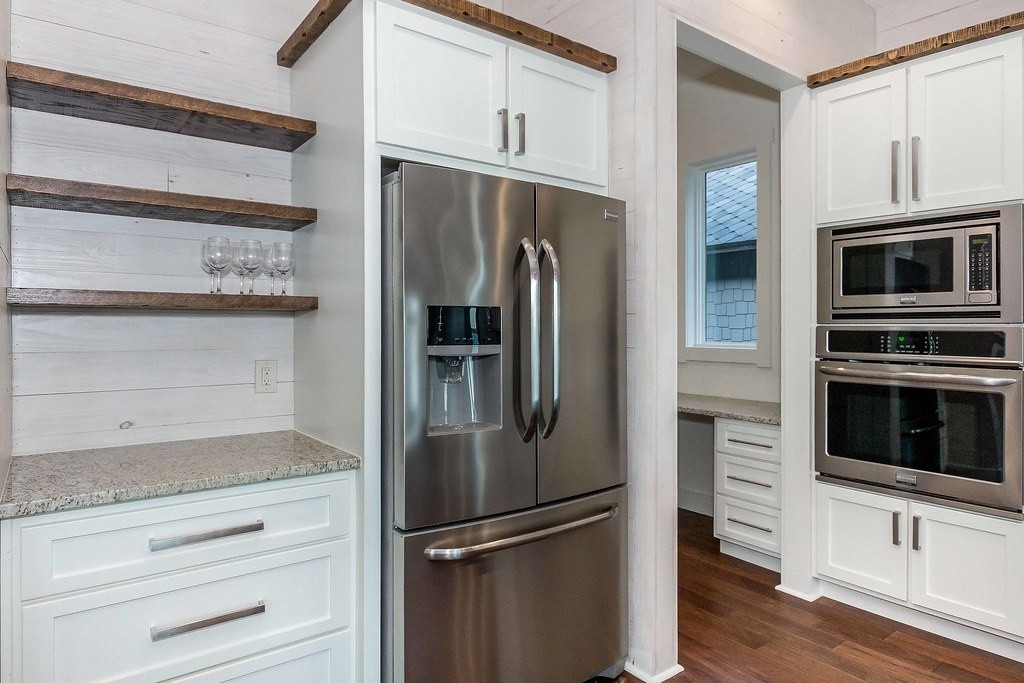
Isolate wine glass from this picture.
[201,237,294,296]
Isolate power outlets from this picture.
[255,360,278,393]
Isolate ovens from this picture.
[815,203,1024,521]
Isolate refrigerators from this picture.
[383,164,629,683]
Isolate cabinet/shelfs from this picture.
[274,0,620,683]
[0,472,361,683]
[813,481,1024,664]
[5,62,318,315]
[712,415,783,574]
[808,10,1024,231]
